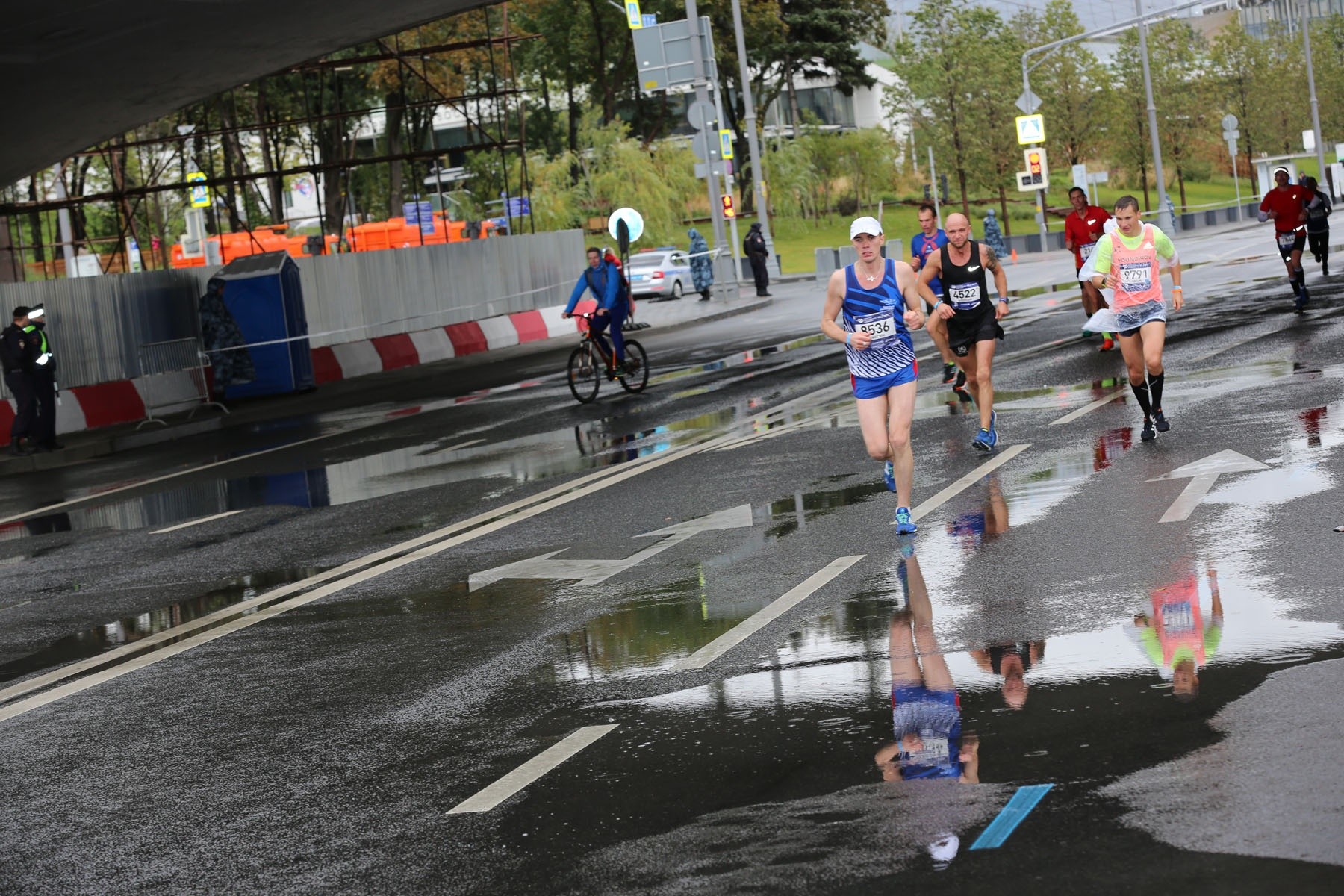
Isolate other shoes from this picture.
[1315,246,1321,262]
[1299,284,1309,303]
[1294,295,1305,311]
[1322,263,1329,276]
[699,296,710,301]
[37,441,65,449]
[757,291,772,296]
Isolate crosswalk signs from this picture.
[185,171,212,209]
[718,128,734,160]
[624,0,644,30]
[1015,114,1046,146]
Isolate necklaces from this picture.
[858,261,882,281]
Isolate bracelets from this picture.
[933,299,944,310]
[894,740,903,754]
[1171,281,1182,293]
[846,332,852,345]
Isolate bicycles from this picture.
[566,308,649,404]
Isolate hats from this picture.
[27,303,45,319]
[751,222,762,228]
[13,306,31,317]
[930,832,959,871]
[1273,166,1290,175]
[850,216,883,241]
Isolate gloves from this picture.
[36,352,52,365]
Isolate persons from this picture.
[743,222,773,296]
[561,247,634,377]
[918,212,1008,452]
[1122,556,1225,708]
[1296,169,1332,276]
[0,303,66,456]
[966,634,1043,713]
[872,541,979,874]
[911,204,969,390]
[1078,195,1183,443]
[820,216,924,535]
[197,276,256,404]
[1064,187,1120,353]
[1258,166,1320,307]
[687,227,713,301]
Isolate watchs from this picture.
[997,296,1009,304]
[1101,277,1107,287]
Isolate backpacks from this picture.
[587,262,629,300]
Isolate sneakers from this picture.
[1151,405,1170,432]
[973,410,998,452]
[604,365,620,371]
[942,362,957,383]
[1100,338,1114,351]
[952,370,967,390]
[1113,333,1119,341]
[883,460,897,493]
[895,507,918,535]
[1141,417,1156,441]
[615,371,629,376]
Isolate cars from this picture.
[624,246,698,300]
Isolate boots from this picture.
[12,435,25,455]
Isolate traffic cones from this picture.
[1011,248,1018,261]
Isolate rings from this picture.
[1110,282,1113,286]
[862,342,868,347]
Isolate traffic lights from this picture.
[721,194,737,221]
[1015,147,1049,193]
